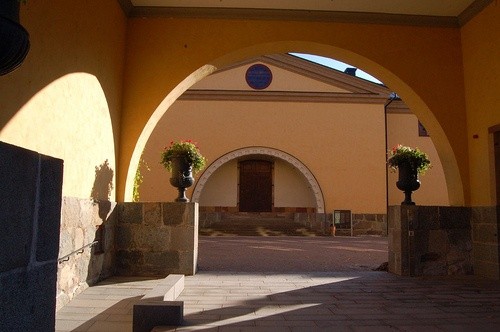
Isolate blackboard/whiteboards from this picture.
[332,209,352,231]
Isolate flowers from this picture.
[385,144,431,173]
[160,138,206,175]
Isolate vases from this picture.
[396,164,421,205]
[169,158,194,201]
